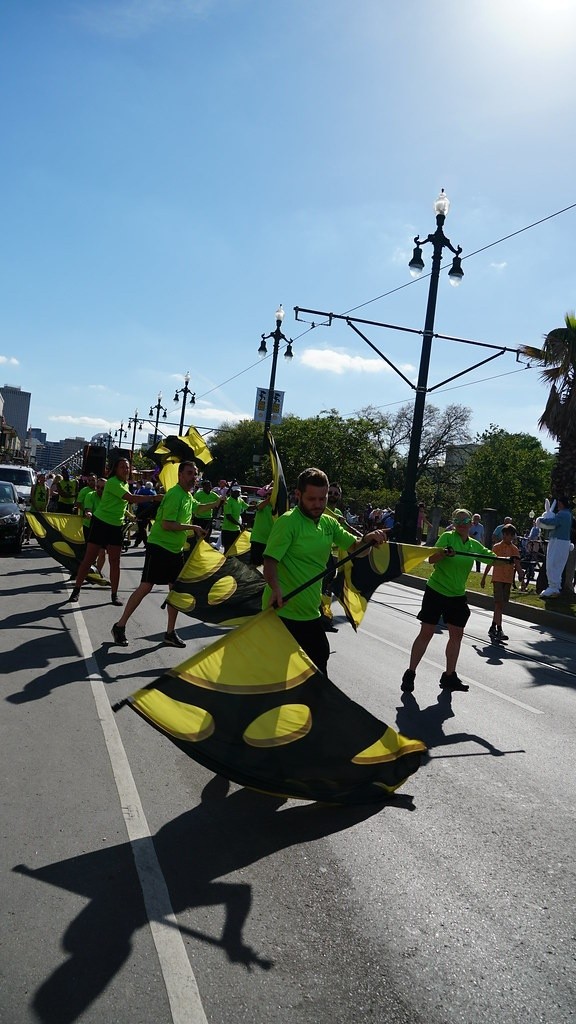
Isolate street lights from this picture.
[115,418,127,448]
[392,189,465,544]
[148,391,167,446]
[257,304,294,488]
[172,370,196,437]
[50,428,116,478]
[128,408,142,493]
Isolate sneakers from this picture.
[112,593,123,606]
[488,625,499,638]
[68,587,80,602]
[109,622,129,646]
[400,668,417,693]
[163,630,187,649]
[438,670,470,691]
[491,629,509,640]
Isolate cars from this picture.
[0,481,28,556]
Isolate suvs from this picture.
[0,464,37,517]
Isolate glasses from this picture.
[96,484,105,490]
[87,479,97,483]
[452,517,474,526]
[328,490,341,497]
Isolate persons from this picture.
[401,510,521,691]
[523,518,540,559]
[467,512,485,572]
[31,469,433,576]
[110,461,227,648]
[480,525,524,639]
[261,468,393,677]
[492,515,518,548]
[535,494,575,597]
[66,457,167,607]
[320,480,362,623]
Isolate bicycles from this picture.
[513,540,548,591]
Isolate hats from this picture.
[232,485,242,492]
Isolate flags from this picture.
[160,534,267,627]
[264,425,294,523]
[143,425,214,493]
[21,511,111,586]
[123,602,433,807]
[334,534,447,632]
